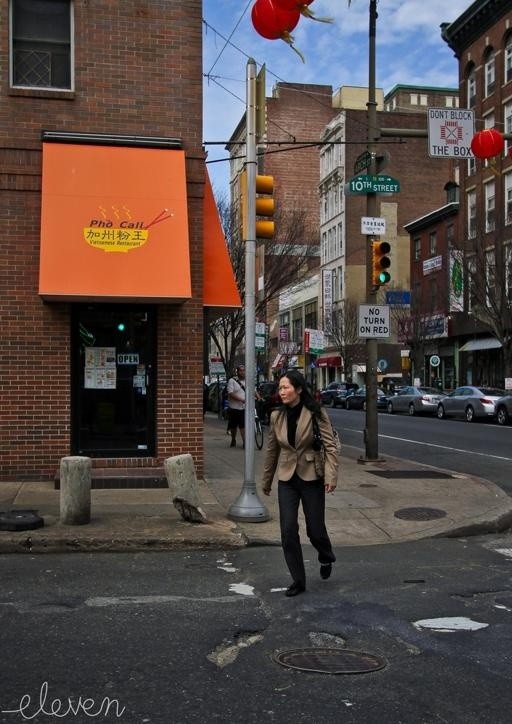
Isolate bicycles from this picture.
[255,398,266,451]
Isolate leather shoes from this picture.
[320,562,331,579]
[285,583,306,597]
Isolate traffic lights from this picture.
[372,240,391,288]
[240,170,277,241]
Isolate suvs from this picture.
[320,382,359,409]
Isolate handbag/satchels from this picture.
[313,430,343,478]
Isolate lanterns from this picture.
[278,0,336,24]
[471,128,504,176]
[251,0,305,64]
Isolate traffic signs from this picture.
[360,215,387,236]
[305,328,324,356]
[359,304,391,337]
[348,175,401,194]
[427,105,477,162]
[255,322,267,353]
[354,151,371,174]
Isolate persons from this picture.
[227,365,261,449]
[203,380,209,420]
[262,372,339,597]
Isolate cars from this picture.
[495,392,512,425]
[385,386,446,416]
[437,385,507,422]
[207,381,321,424]
[345,386,399,411]
